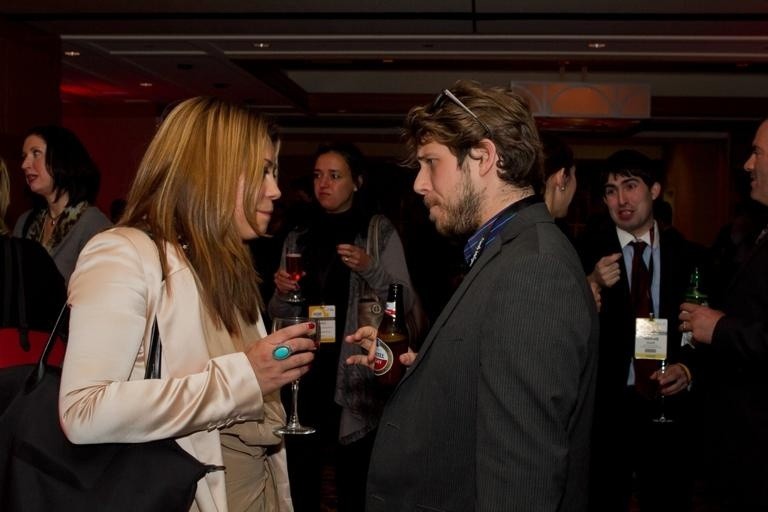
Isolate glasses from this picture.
[429,88,491,138]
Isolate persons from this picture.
[531,131,604,317]
[57,95,319,512]
[1,162,70,415]
[268,140,416,512]
[342,79,602,511]
[582,147,703,509]
[678,118,768,511]
[10,122,115,291]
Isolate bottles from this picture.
[372,282,409,400]
[681,263,708,306]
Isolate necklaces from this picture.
[47,211,61,226]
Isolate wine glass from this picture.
[275,235,307,304]
[650,357,676,425]
[268,315,322,437]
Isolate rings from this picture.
[682,321,687,332]
[272,343,293,361]
[345,256,350,262]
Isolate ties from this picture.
[628,242,657,397]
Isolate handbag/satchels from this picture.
[1,355,225,512]
[0,318,62,368]
[355,289,418,352]
